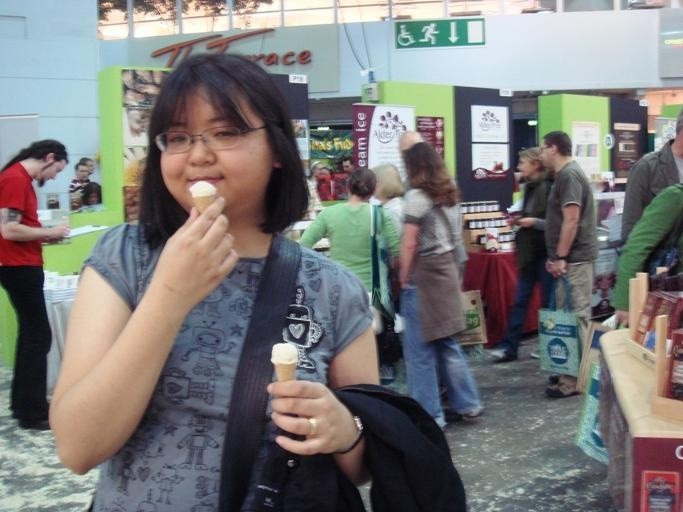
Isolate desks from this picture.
[463,250,543,349]
[598,328,683,512]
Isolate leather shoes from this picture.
[19,419,50,430]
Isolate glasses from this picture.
[154,124,266,154]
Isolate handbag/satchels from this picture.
[576,361,625,466]
[453,290,489,346]
[370,296,397,358]
[575,321,618,393]
[538,307,586,377]
[615,237,680,276]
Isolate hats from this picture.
[400,131,424,153]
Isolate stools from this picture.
[626,266,683,371]
[649,314,683,421]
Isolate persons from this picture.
[47,52,381,510]
[1,140,104,429]
[608,183,683,327]
[491,145,558,363]
[620,109,683,243]
[297,132,485,430]
[539,131,599,399]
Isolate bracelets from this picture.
[554,255,569,260]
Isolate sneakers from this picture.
[531,350,541,358]
[488,349,518,362]
[546,383,581,397]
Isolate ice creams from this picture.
[188,180,216,214]
[271,343,299,380]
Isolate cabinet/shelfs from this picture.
[461,211,516,254]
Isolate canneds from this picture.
[459,200,500,213]
[465,215,508,230]
[477,231,516,244]
